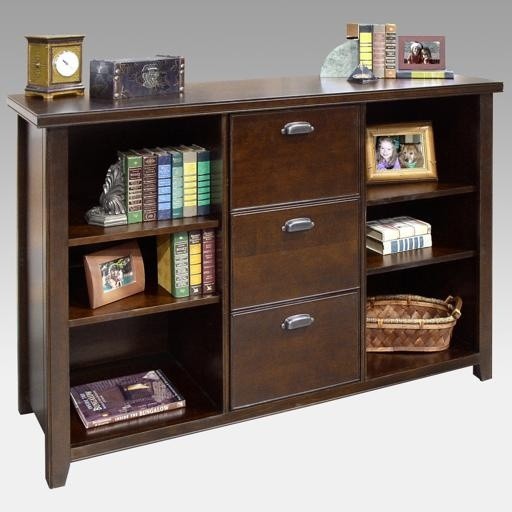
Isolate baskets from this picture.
[366,293,463,353]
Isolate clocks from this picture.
[24,34,85,102]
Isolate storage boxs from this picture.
[89,50,186,101]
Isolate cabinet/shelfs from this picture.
[6,68,505,488]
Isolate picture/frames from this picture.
[364,119,438,186]
[83,241,145,309]
[397,35,446,71]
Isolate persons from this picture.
[104,263,122,289]
[376,139,400,171]
[406,42,431,64]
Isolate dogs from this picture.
[398,143,424,169]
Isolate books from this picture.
[66,368,188,430]
[118,144,211,226]
[200,226,218,295]
[364,234,433,256]
[370,24,387,80]
[384,23,397,79]
[346,23,373,75]
[364,214,433,241]
[184,230,204,298]
[156,234,191,297]
